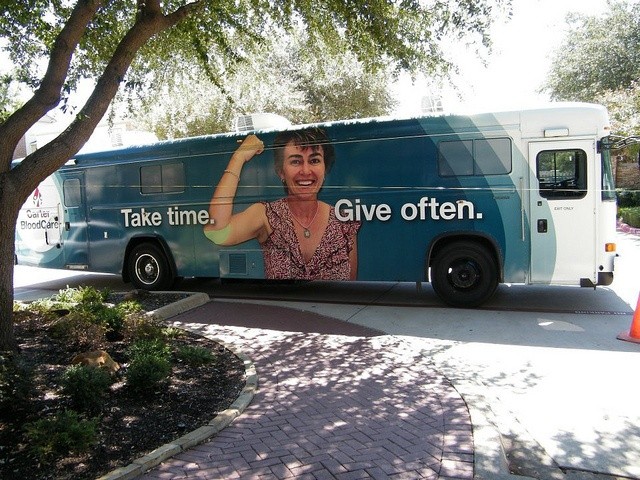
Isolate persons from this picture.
[202,132,364,282]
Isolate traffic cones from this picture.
[616,289,640,345]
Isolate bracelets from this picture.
[223,169,240,181]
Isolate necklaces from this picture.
[288,201,320,237]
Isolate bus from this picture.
[10,102,619,308]
